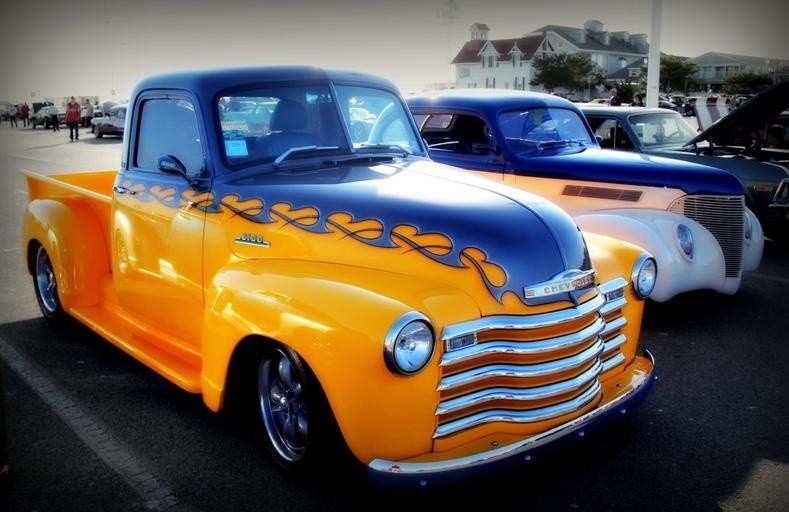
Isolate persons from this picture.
[608,85,695,117]
[7,96,100,143]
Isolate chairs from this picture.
[257,100,324,158]
